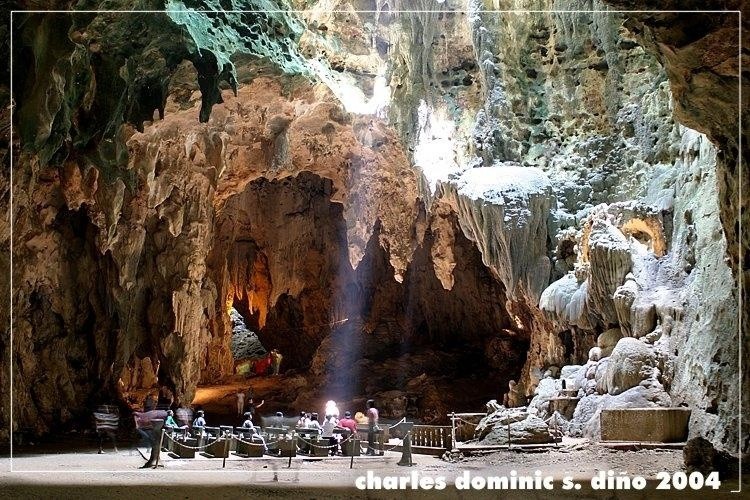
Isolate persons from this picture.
[164,410,191,437]
[192,410,212,440]
[240,396,381,457]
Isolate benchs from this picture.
[173,426,360,459]
